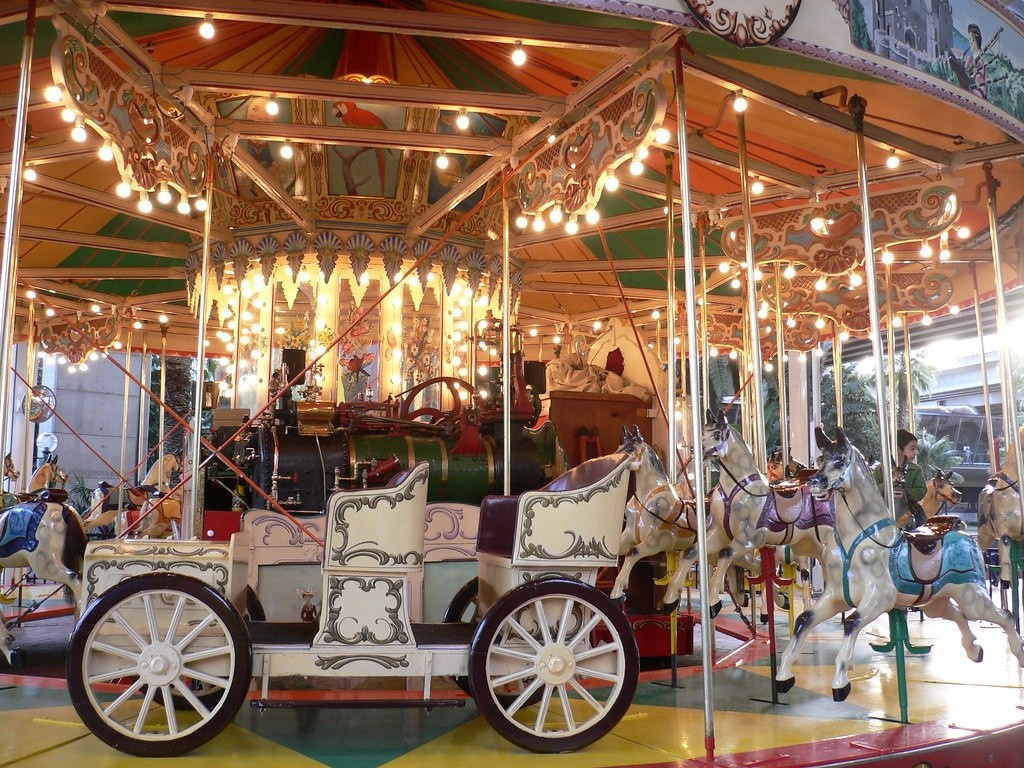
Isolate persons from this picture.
[872,429,929,529]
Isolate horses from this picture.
[917,468,964,519]
[0,488,91,670]
[4,449,21,482]
[976,423,1024,590]
[82,449,184,532]
[660,408,835,619]
[0,454,69,508]
[773,423,1023,704]
[885,453,916,533]
[763,445,806,484]
[125,490,182,540]
[610,422,790,622]
